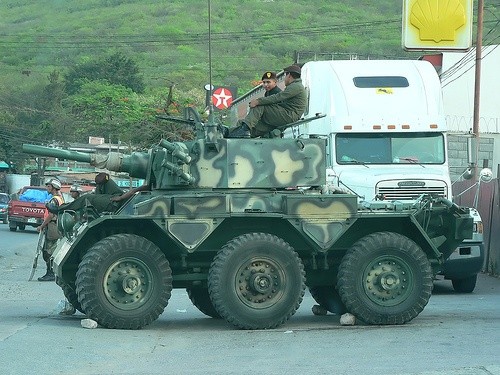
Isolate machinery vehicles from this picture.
[21,0,474,330]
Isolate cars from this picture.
[0,192,11,225]
[7,186,53,233]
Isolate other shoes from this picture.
[45,202,63,216]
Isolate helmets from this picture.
[70,184,83,192]
[45,178,62,190]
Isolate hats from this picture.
[283,66,301,75]
[95,172,110,185]
[261,71,276,81]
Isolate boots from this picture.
[38,267,55,281]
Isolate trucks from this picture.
[280,58,455,207]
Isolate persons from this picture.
[69,184,86,201]
[47,176,151,214]
[223,65,308,138]
[37,179,65,281]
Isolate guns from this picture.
[155,115,196,126]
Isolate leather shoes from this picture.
[223,125,251,139]
